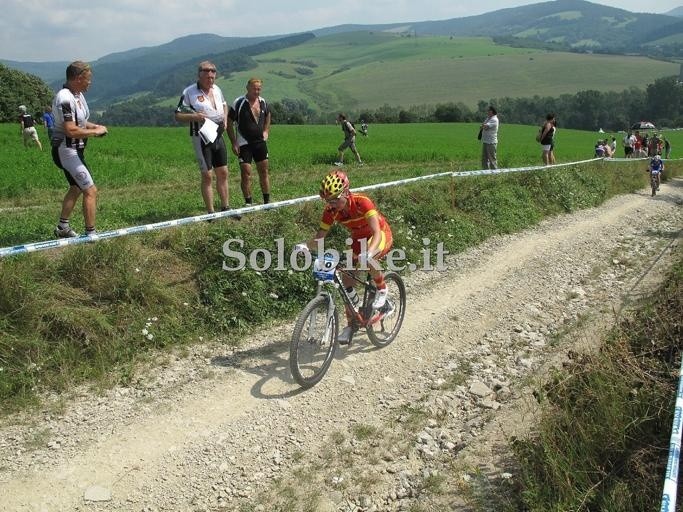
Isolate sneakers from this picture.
[372,286,388,309]
[337,326,353,344]
[54,224,77,238]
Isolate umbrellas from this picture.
[629,121,656,138]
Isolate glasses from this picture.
[201,68,216,73]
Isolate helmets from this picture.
[319,171,349,202]
[654,155,661,162]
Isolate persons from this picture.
[226,77,276,212]
[43,106,54,143]
[359,120,368,136]
[18,105,43,152]
[174,61,242,222]
[540,113,557,166]
[479,106,499,170]
[334,112,364,167]
[592,130,671,159]
[294,170,392,342]
[50,60,109,238]
[647,155,664,191]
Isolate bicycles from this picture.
[289,250,405,389]
[645,168,663,197]
